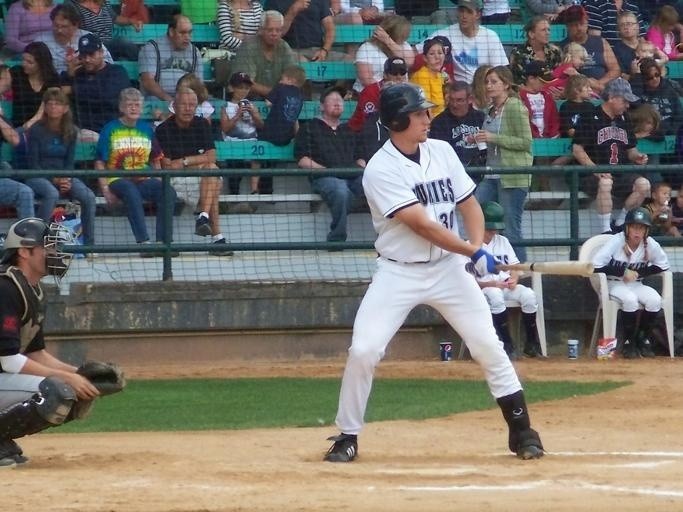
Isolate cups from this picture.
[439,342,452,361]
[658,200,668,221]
[237,99,250,121]
[472,133,488,152]
[567,340,579,360]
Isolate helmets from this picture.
[380,83,436,131]
[0,217,73,289]
[481,201,505,230]
[625,207,652,238]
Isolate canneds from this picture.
[239,99,251,121]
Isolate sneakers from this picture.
[621,337,655,359]
[324,433,357,462]
[509,429,543,459]
[0,439,29,468]
[524,343,542,356]
[195,212,234,255]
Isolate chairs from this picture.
[578,234,674,359]
[458,270,547,360]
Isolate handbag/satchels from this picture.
[51,217,86,257]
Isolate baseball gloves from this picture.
[78,361,126,396]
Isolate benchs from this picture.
[0,0,683,213]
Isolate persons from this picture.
[592,207,671,360]
[465,199,546,361]
[0,216,125,470]
[1,0,683,257]
[324,82,546,463]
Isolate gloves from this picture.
[468,249,501,277]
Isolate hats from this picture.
[76,33,101,53]
[603,76,639,102]
[322,87,347,100]
[523,60,558,83]
[458,0,483,11]
[384,56,406,72]
[231,72,253,85]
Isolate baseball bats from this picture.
[465,262,595,278]
